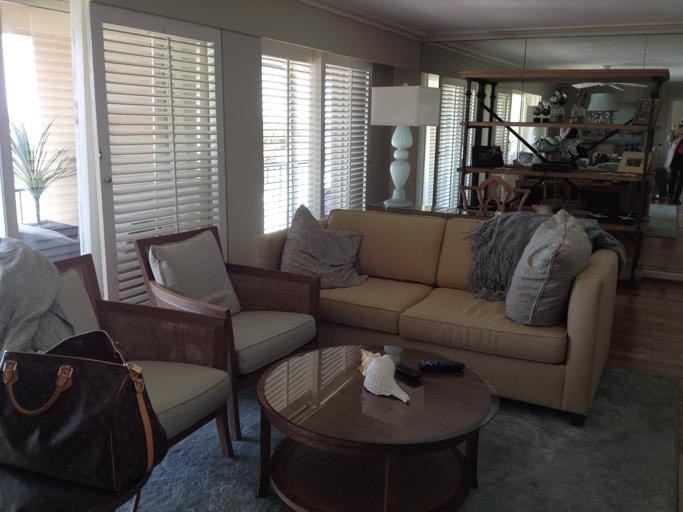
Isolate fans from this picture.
[571,65,648,92]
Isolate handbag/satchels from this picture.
[1,324,171,491]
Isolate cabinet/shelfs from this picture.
[457,68,670,288]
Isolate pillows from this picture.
[280,205,368,288]
[504,209,592,327]
[57,266,100,336]
[147,230,241,316]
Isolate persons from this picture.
[663,121,682,205]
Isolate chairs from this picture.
[0,253,234,461]
[134,226,321,440]
[460,175,530,213]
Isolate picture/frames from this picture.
[618,151,652,176]
[632,99,662,128]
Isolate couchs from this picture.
[254,209,620,415]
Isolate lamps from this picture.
[370,83,441,208]
[586,93,618,135]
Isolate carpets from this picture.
[117,367,683,512]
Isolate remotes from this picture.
[393,362,423,380]
[418,360,465,374]
